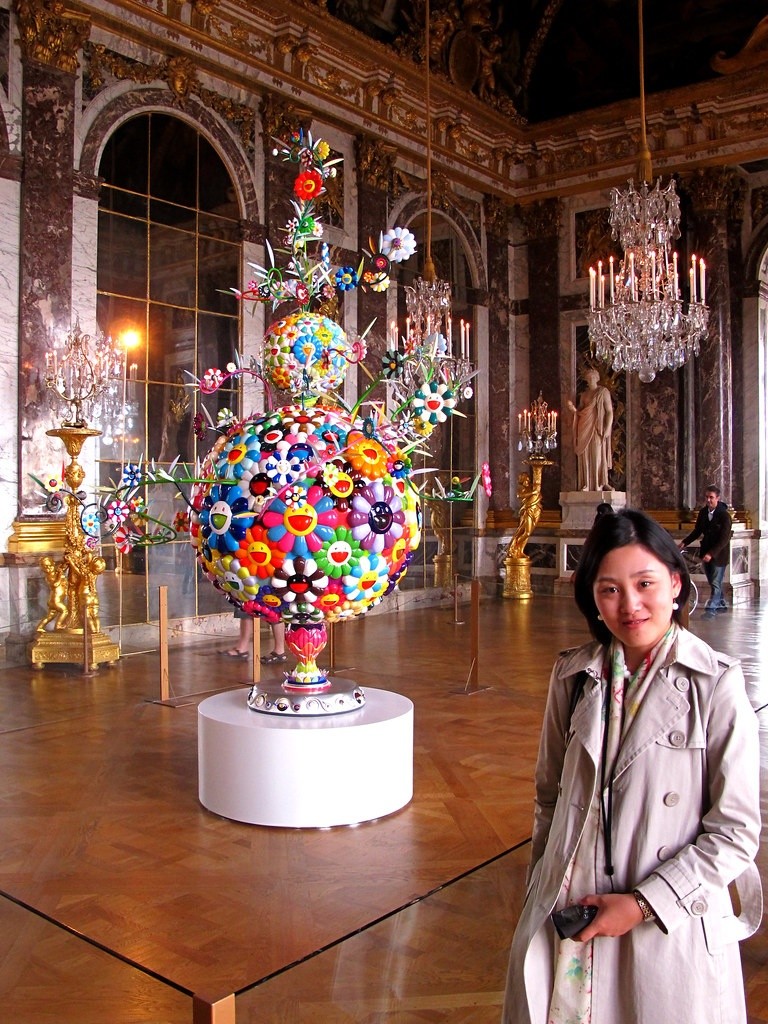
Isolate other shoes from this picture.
[705,605,727,612]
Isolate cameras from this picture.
[550,903,598,940]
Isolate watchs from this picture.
[634,891,656,922]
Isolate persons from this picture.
[679,486,731,617]
[425,474,457,554]
[221,607,289,664]
[510,472,542,558]
[36,553,107,634]
[422,18,501,100]
[567,370,615,491]
[503,502,764,1024]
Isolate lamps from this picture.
[389,0,473,403]
[587,0,712,384]
[44,314,112,429]
[517,388,558,456]
[101,344,139,450]
